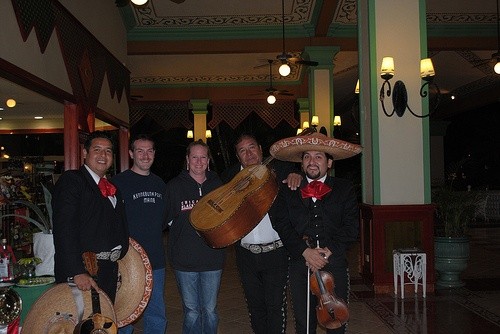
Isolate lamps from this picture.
[312,115,343,138]
[379,56,442,117]
[493,58,500,74]
[266,92,276,104]
[278,59,291,76]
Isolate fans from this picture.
[248,59,294,98]
[254,0,320,69]
[472,0,500,68]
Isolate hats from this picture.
[270,128,362,161]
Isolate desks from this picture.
[393,250,427,299]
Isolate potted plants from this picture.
[0,183,60,278]
[432,191,481,288]
[13,258,56,327]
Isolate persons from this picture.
[109,134,168,334]
[267,132,362,334]
[167,142,227,334]
[222,133,303,334]
[50,130,129,305]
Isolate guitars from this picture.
[189,125,318,249]
[73,251,117,334]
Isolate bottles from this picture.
[0,238,13,281]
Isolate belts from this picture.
[96,250,122,262]
[240,239,284,254]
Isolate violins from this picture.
[302,235,350,329]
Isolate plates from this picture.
[15,277,57,287]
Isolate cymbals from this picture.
[0,286,23,326]
